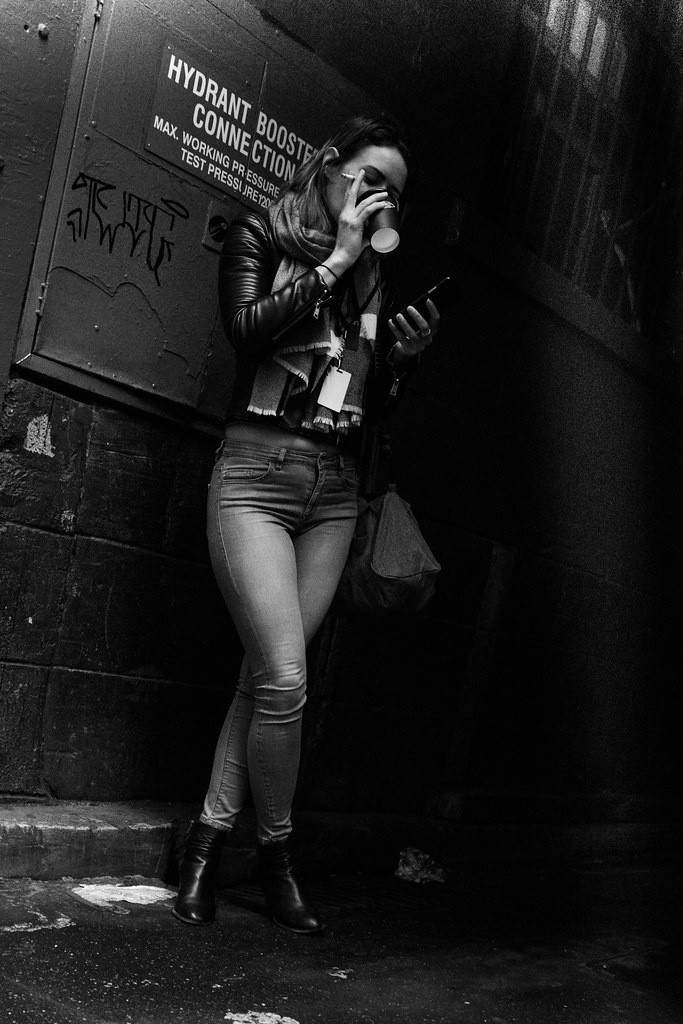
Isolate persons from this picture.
[165,114,449,937]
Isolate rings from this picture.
[420,327,432,338]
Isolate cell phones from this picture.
[395,277,454,333]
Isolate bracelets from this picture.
[318,265,339,281]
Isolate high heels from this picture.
[162,819,231,925]
[257,832,326,934]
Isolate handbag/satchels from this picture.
[343,483,440,615]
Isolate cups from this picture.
[356,188,402,253]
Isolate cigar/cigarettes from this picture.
[341,173,356,180]
